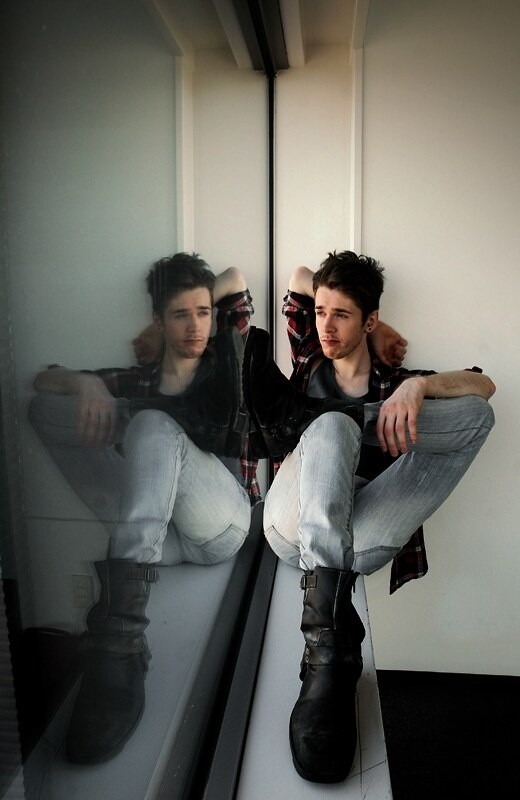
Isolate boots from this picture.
[288,567,365,783]
[48,557,157,764]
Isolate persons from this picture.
[5,251,288,768]
[265,249,495,785]
